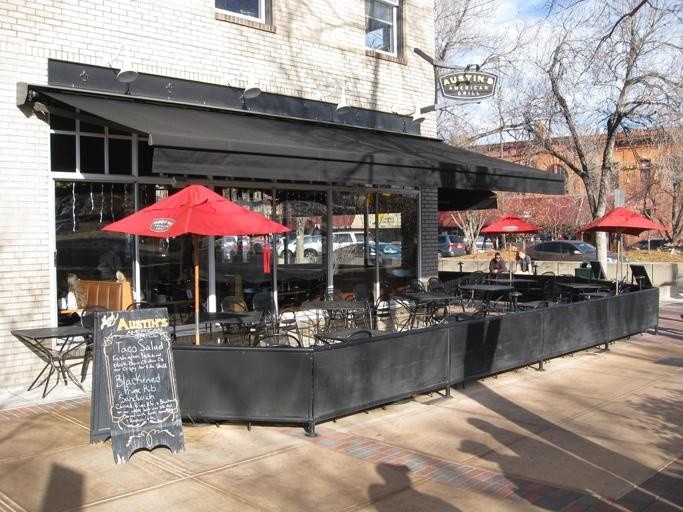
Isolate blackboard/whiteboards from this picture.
[97,308,185,465]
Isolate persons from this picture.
[489,252,507,273]
[513,249,532,275]
[57,272,87,326]
[96,241,123,280]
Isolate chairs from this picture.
[76,270,635,432]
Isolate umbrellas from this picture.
[575,207,672,296]
[479,215,540,286]
[101,184,290,346]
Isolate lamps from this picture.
[412,92,425,124]
[334,84,351,114]
[116,43,138,85]
[242,62,260,100]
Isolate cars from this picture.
[514,240,617,265]
[220,230,496,262]
[631,238,671,251]
